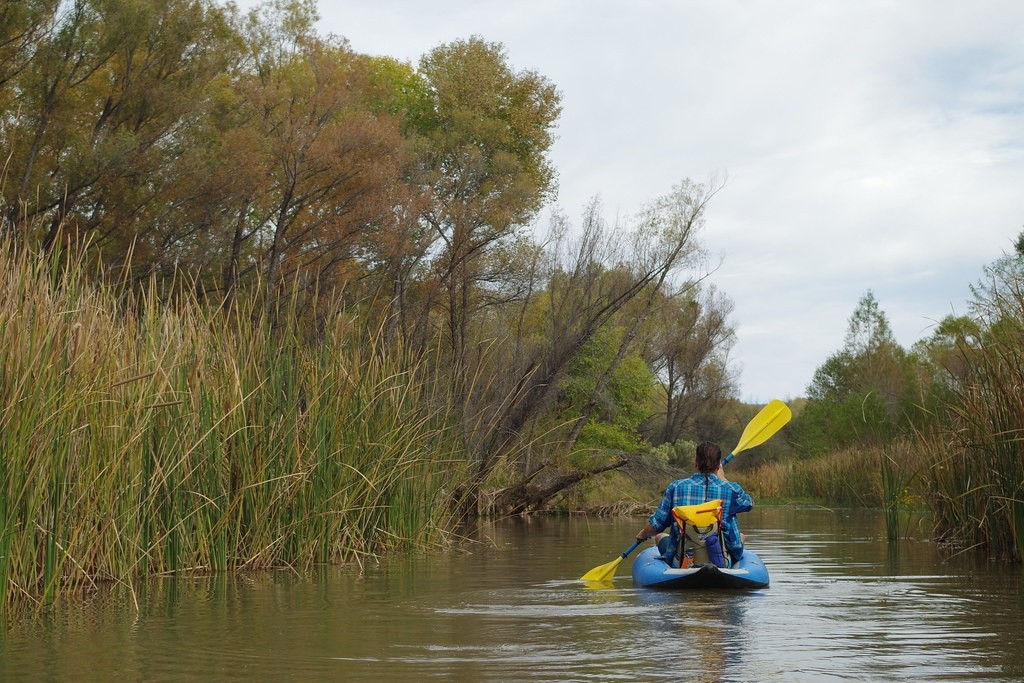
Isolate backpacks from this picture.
[672,499,728,569]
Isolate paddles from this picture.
[581,399,793,581]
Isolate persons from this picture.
[636,442,753,568]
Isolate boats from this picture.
[632,498,770,589]
